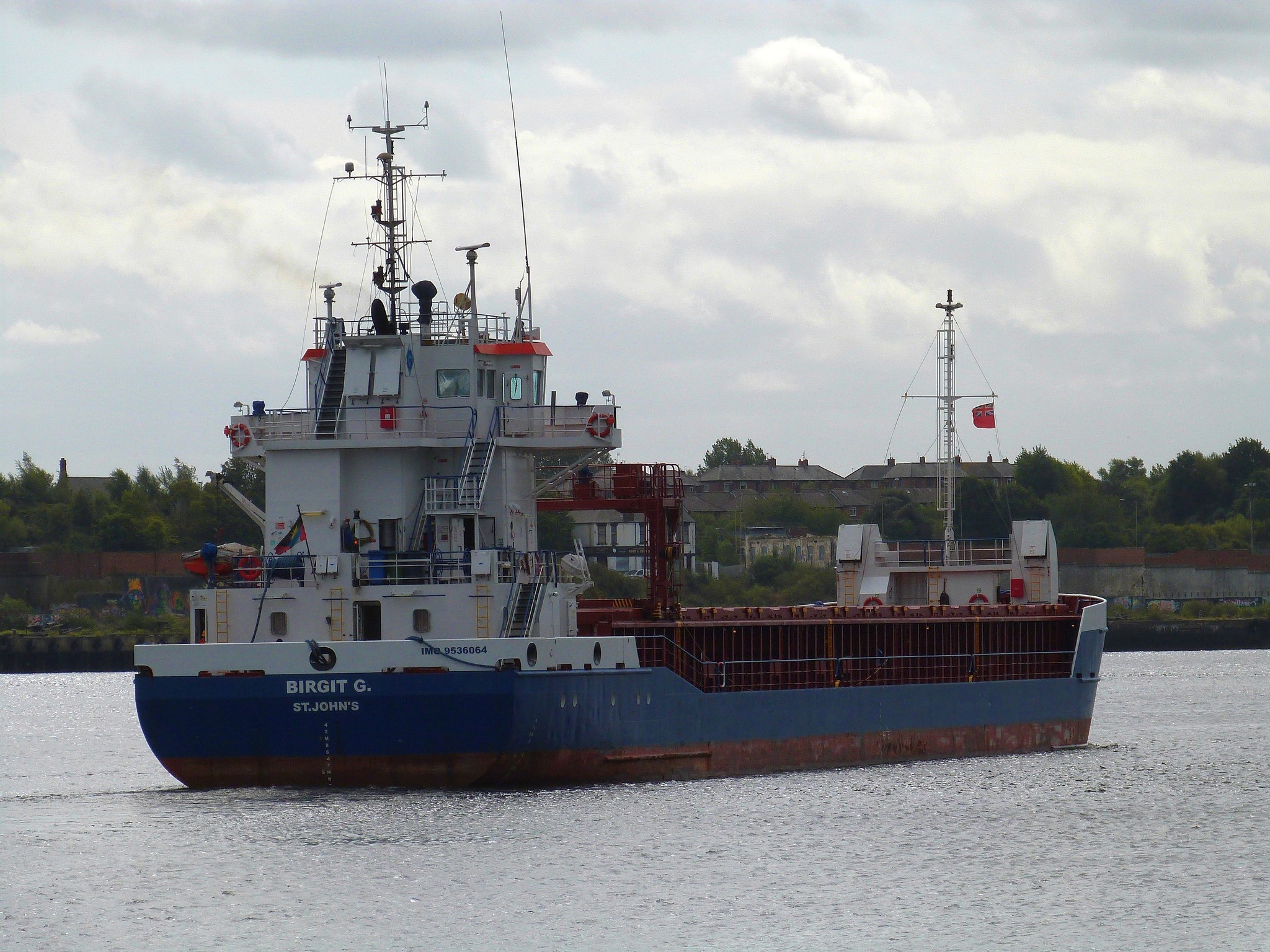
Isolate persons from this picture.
[198,631,206,643]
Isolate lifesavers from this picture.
[969,594,988,603]
[238,556,262,581]
[588,414,611,437]
[231,423,250,448]
[863,596,883,606]
[537,554,542,576]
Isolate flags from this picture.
[971,402,996,428]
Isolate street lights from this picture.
[882,478,899,538]
[1244,483,1256,555]
[1120,499,1138,548]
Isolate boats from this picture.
[132,11,1112,791]
[180,542,258,577]
